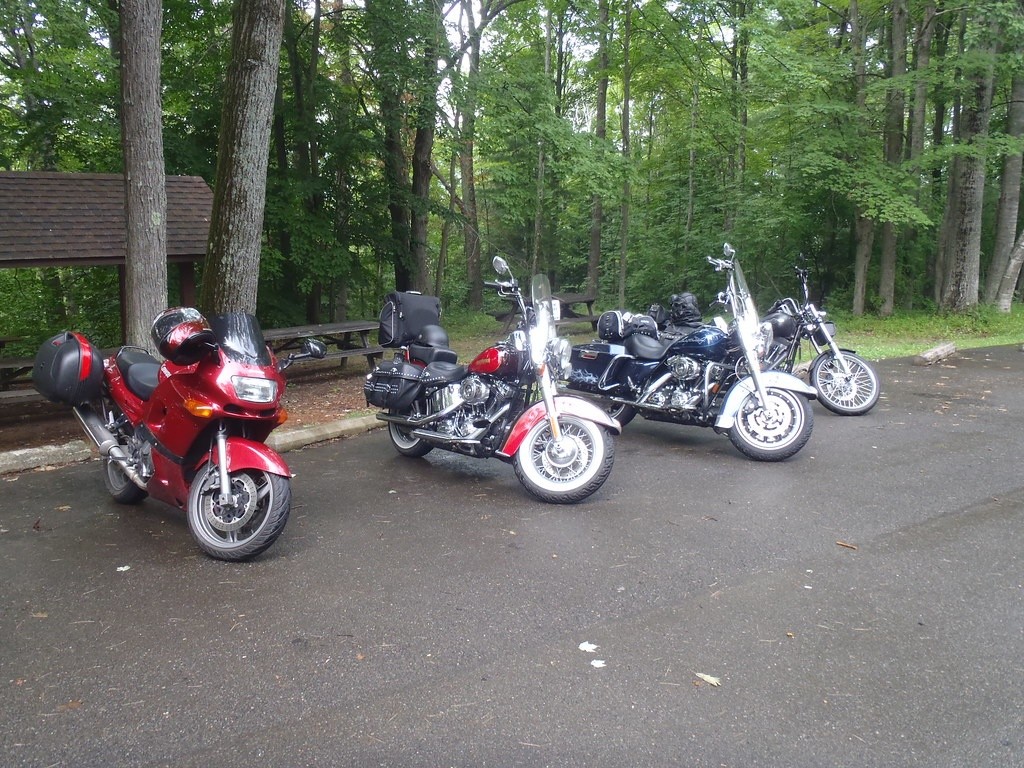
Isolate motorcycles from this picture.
[556,242,882,462]
[362,256,623,504]
[31,307,327,561]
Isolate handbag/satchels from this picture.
[597,310,660,340]
[670,293,701,323]
[377,291,442,348]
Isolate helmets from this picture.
[150,306,216,365]
